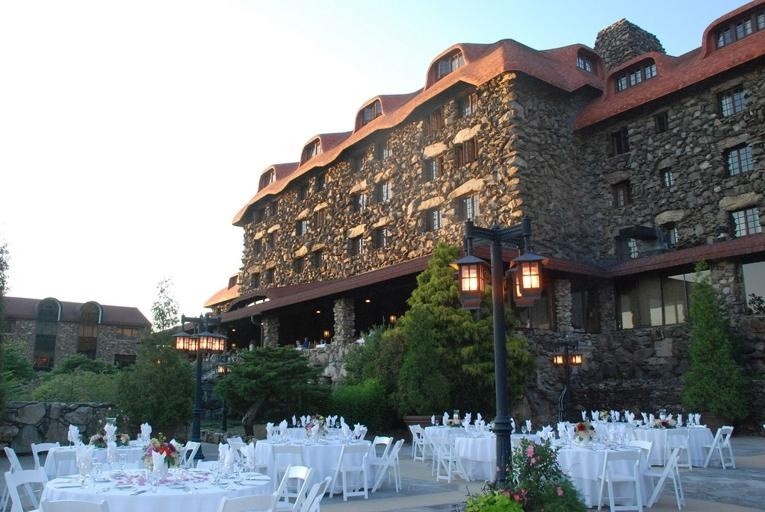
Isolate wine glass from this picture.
[57,432,151,449]
[260,427,368,447]
[49,455,257,496]
[428,410,530,437]
[578,412,636,423]
[637,418,708,430]
[535,426,644,447]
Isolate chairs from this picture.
[299,476,331,511]
[4,467,49,511]
[0,407,653,471]
[642,447,685,510]
[275,464,313,507]
[435,438,470,483]
[700,426,737,472]
[40,497,109,510]
[370,438,406,494]
[596,448,643,512]
[328,443,372,501]
[662,427,694,472]
[221,491,277,511]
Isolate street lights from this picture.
[552,338,583,422]
[214,354,235,431]
[173,312,230,459]
[456,213,551,490]
